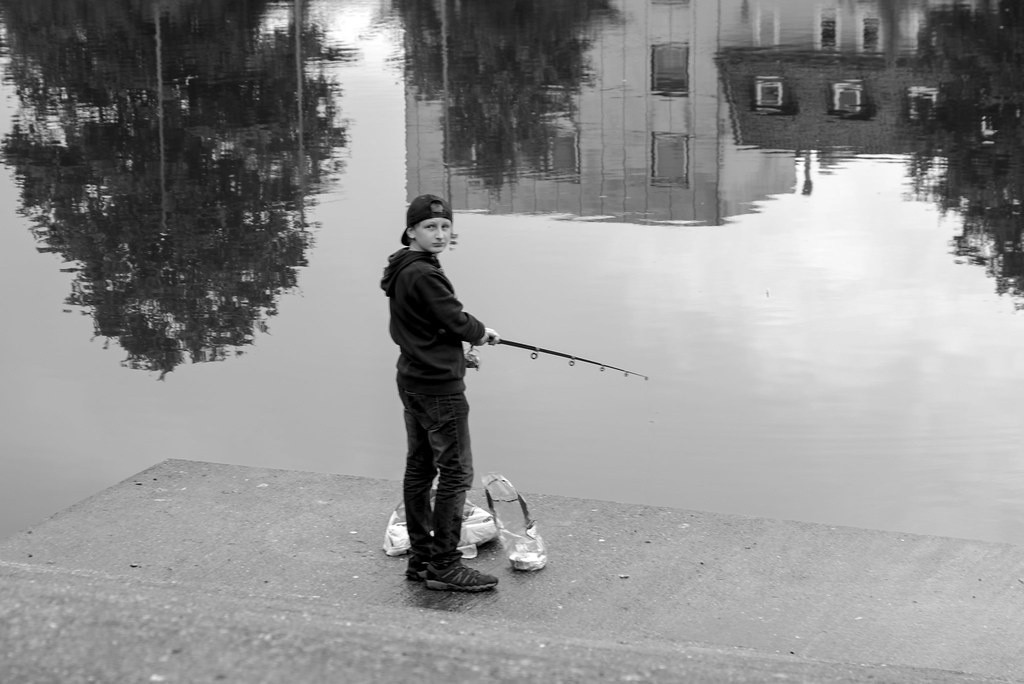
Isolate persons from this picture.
[379,193,501,589]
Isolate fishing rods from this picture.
[464,337,651,382]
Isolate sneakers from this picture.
[405,554,498,590]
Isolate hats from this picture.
[401,194,452,246]
